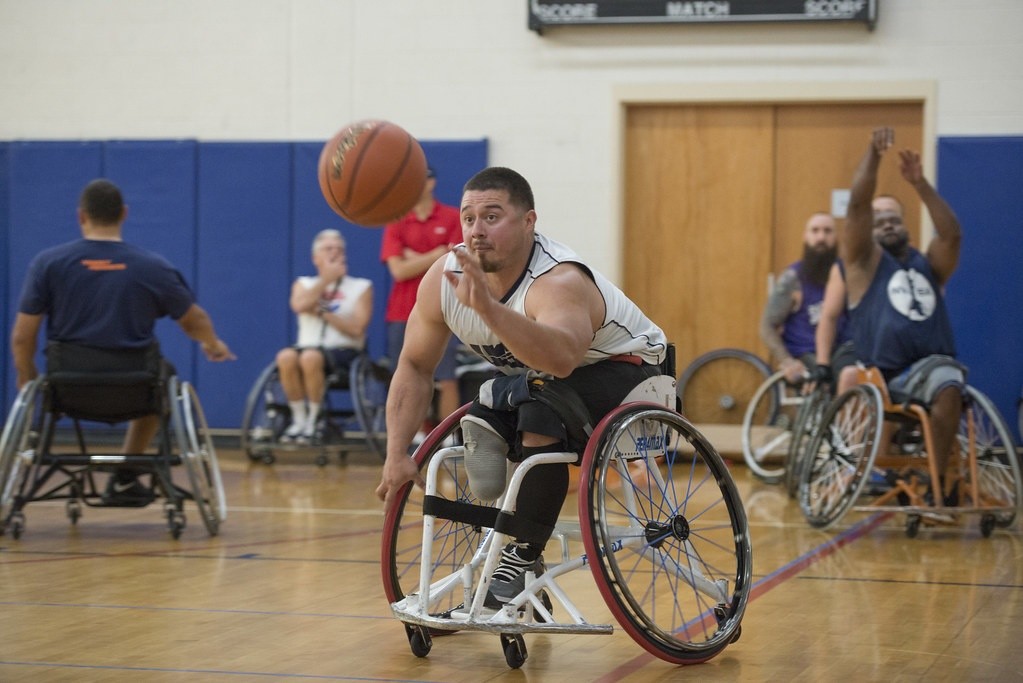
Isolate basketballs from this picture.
[318,118,428,227]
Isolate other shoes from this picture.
[439,433,461,449]
[277,417,306,444]
[924,490,953,508]
[296,417,330,445]
[411,415,436,446]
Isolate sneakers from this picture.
[472,537,545,610]
[102,476,158,504]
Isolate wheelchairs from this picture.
[381,342,753,669]
[241,337,395,466]
[0,340,226,540]
[742,339,1022,539]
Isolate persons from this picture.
[375,166,669,622]
[377,159,466,469]
[275,227,374,448]
[756,123,965,509]
[10,179,239,504]
